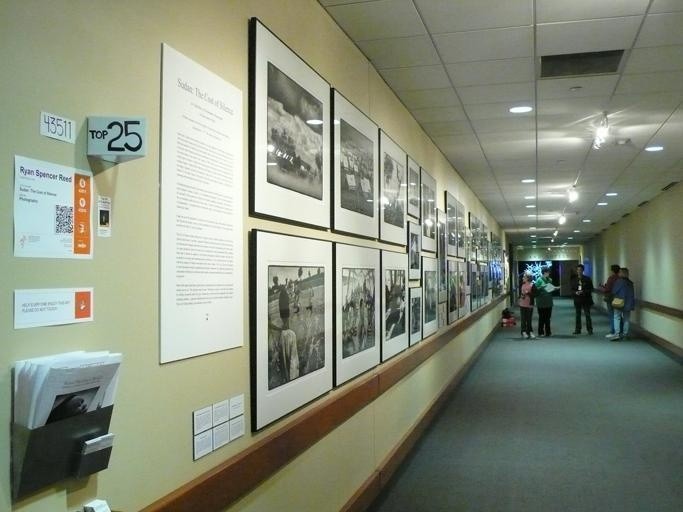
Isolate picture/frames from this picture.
[407,155,420,219]
[381,248,409,361]
[332,240,381,387]
[378,128,409,246]
[444,190,457,257]
[458,201,466,258]
[251,228,330,432]
[422,256,438,340]
[420,167,438,252]
[447,260,459,325]
[456,263,469,318]
[249,18,333,233]
[409,287,422,346]
[330,87,380,243]
[469,215,506,311]
[407,221,421,280]
[436,209,448,303]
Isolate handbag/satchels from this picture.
[611,297,624,309]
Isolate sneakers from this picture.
[530,331,536,340]
[609,335,622,341]
[605,333,614,338]
[522,331,529,338]
[622,335,628,342]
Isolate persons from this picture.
[598,264,620,338]
[535,268,553,337]
[411,235,418,266]
[341,282,375,355]
[268,278,315,387]
[570,263,594,336]
[397,296,405,326]
[609,267,635,341]
[270,127,323,182]
[518,272,539,339]
